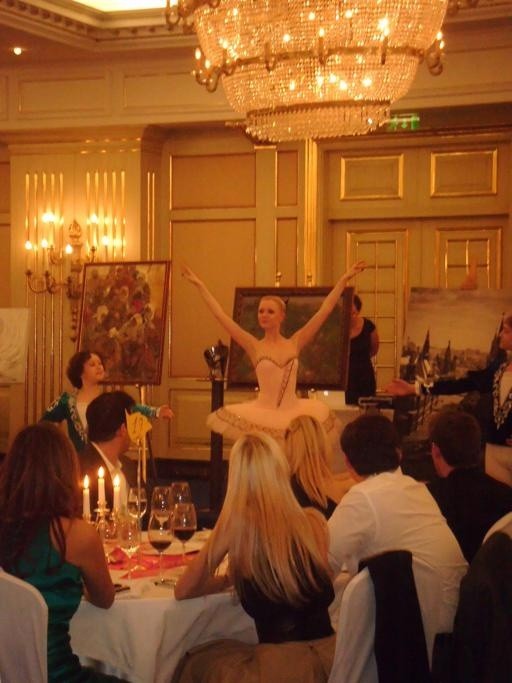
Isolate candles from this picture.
[82,478,122,522]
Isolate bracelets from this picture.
[224,573,231,588]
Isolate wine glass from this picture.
[126,488,147,547]
[147,513,177,584]
[151,487,174,526]
[119,518,141,588]
[171,482,192,504]
[98,526,118,574]
[171,503,198,570]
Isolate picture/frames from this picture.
[76,260,172,385]
[226,285,354,391]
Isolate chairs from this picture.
[0,564,50,683]
[326,512,512,682]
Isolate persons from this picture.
[281,416,338,523]
[0,419,132,683]
[174,430,340,683]
[420,412,512,570]
[35,349,176,462]
[73,390,154,530]
[319,411,471,677]
[177,258,369,457]
[84,264,161,379]
[345,292,382,406]
[379,314,512,485]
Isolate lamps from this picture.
[164,0,448,142]
[24,218,109,301]
